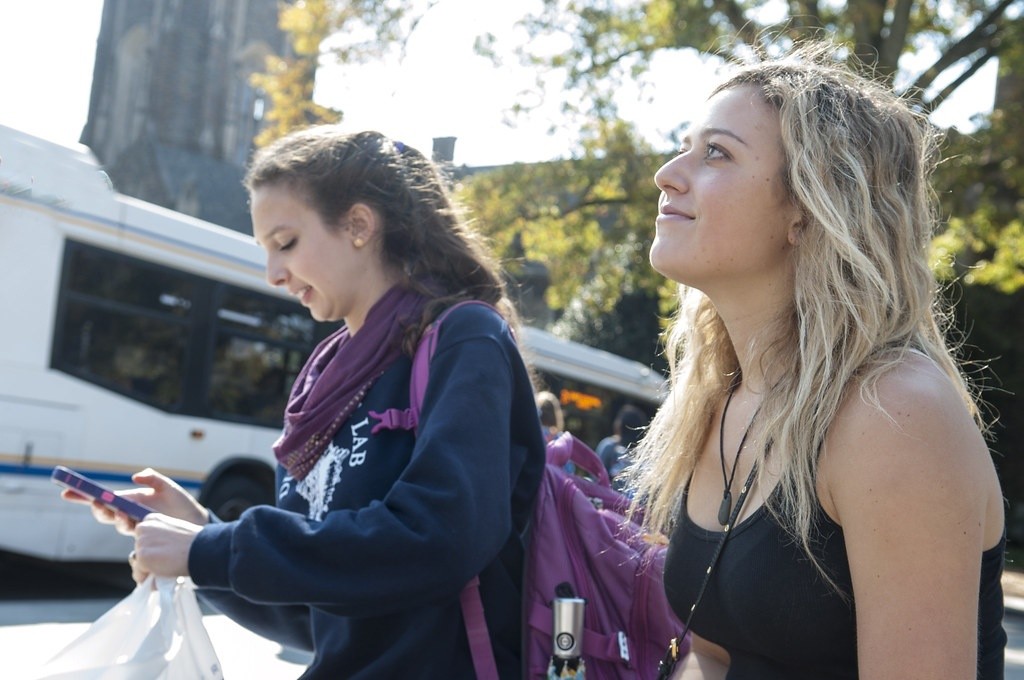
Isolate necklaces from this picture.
[717,373,786,526]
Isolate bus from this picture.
[0,126,672,561]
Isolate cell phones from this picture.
[52,466,155,522]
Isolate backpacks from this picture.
[369,300,691,680]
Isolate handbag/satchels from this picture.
[26,566,225,680]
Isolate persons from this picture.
[612,61,1008,680]
[60,124,547,680]
[536,391,651,499]
